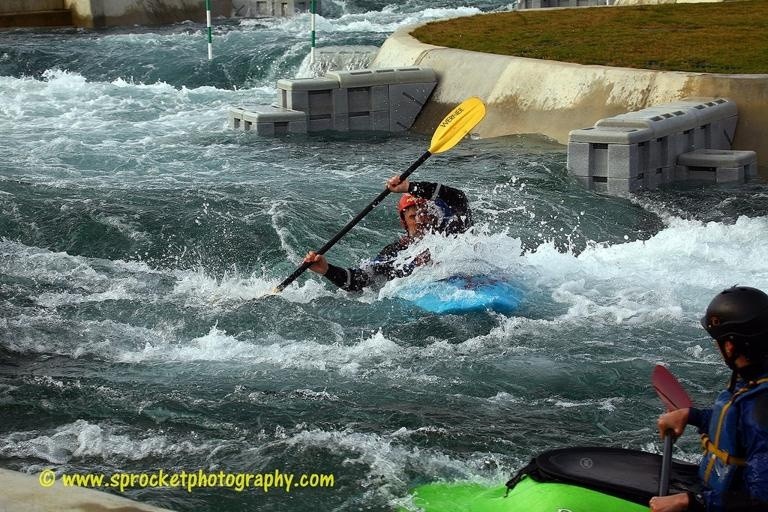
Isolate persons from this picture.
[649,286,767,512]
[305,174,473,293]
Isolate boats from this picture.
[394,446,702,511]
[409,273,524,317]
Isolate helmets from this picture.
[701,285,768,340]
[399,193,428,213]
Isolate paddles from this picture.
[653,364,696,495]
[277,97,485,293]
